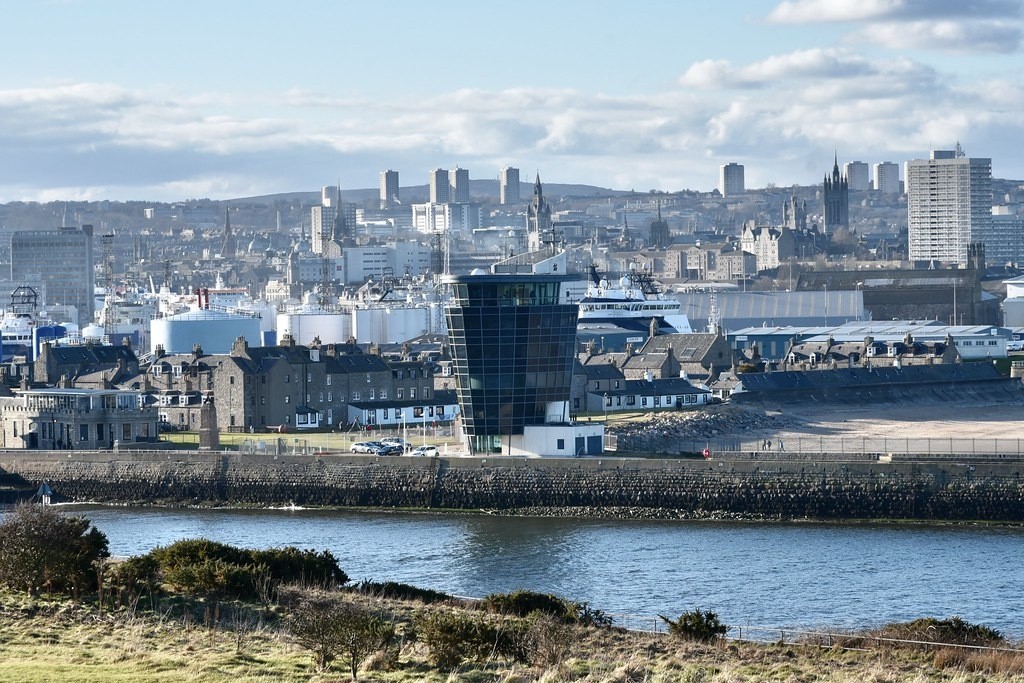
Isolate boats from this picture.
[1,313,34,351]
[576,276,692,353]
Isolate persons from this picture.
[767,440,772,450]
[53,438,72,450]
[762,438,766,450]
[779,440,785,452]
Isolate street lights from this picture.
[853,281,863,322]
[420,411,425,444]
[822,284,827,328]
[925,312,965,326]
[952,277,959,325]
[788,257,791,291]
[400,411,406,453]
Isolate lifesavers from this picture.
[703,449,710,458]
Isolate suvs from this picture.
[374,445,403,456]
[372,438,412,452]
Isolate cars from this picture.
[157,421,179,432]
[409,445,440,457]
[351,442,380,454]
[1008,344,1021,351]
